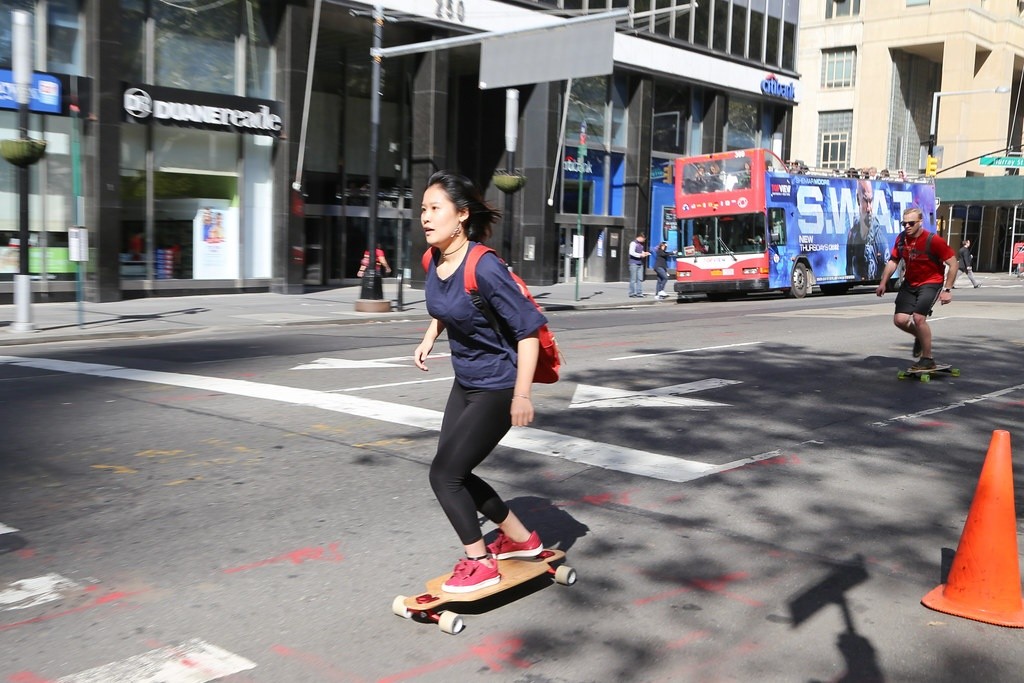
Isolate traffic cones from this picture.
[920,429,1024,628]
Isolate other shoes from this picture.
[658,291,669,297]
[639,295,645,298]
[630,294,638,298]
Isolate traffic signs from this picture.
[980,157,1024,167]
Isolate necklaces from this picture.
[439,237,468,259]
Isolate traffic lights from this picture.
[926,155,937,177]
[662,166,671,184]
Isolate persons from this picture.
[693,232,765,253]
[365,244,391,277]
[845,179,891,282]
[414,169,547,593]
[951,240,982,288]
[833,166,906,181]
[784,159,812,175]
[628,232,651,298]
[876,207,960,370]
[696,162,753,192]
[655,242,677,299]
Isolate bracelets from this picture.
[513,393,531,399]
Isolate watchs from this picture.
[943,287,951,292]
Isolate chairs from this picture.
[718,170,738,191]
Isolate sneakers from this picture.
[485,529,543,559]
[912,357,937,370]
[442,557,500,593]
[912,338,922,358]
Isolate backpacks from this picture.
[422,245,562,384]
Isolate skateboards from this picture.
[897,362,961,382]
[391,547,578,636]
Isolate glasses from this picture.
[900,221,920,226]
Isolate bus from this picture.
[673,148,937,300]
